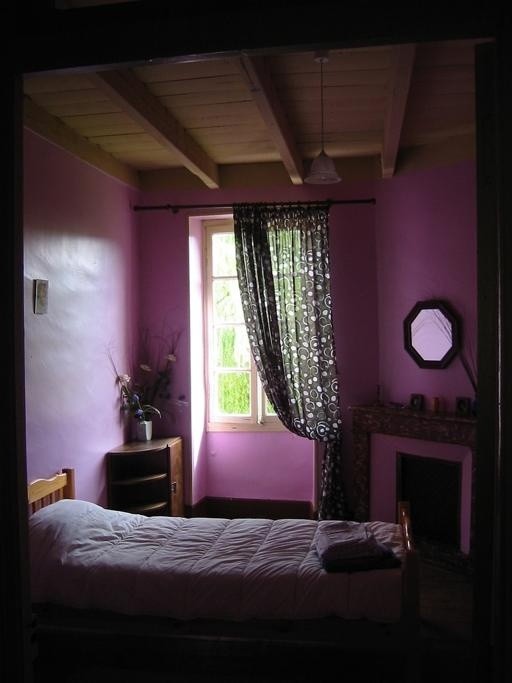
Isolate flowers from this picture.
[110,327,189,425]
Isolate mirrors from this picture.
[402,298,462,369]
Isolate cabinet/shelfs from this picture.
[106,435,185,516]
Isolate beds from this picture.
[25,466,420,682]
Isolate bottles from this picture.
[430,396,439,413]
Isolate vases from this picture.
[136,421,153,440]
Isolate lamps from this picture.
[302,49,343,185]
